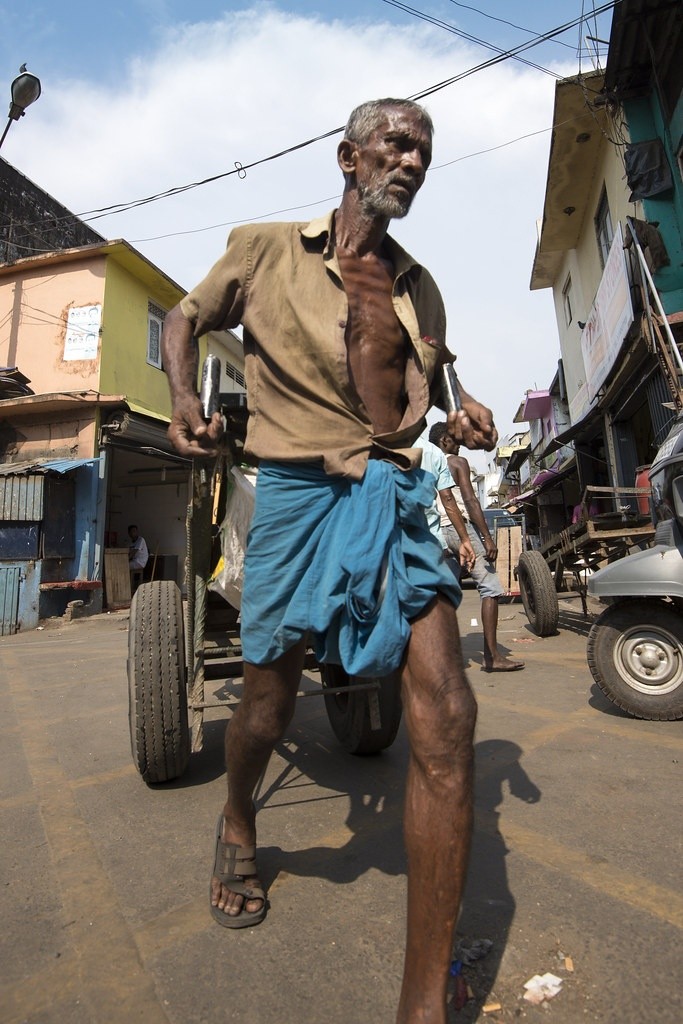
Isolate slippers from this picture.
[209,813,266,928]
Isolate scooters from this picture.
[584,391,683,723]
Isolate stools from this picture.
[131,570,143,596]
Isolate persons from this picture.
[104,524,149,571]
[158,97,500,1024]
[411,420,526,673]
[565,488,600,523]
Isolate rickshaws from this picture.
[117,355,472,791]
[512,485,656,637]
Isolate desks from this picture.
[148,553,179,558]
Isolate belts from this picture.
[442,518,472,528]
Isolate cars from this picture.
[459,507,518,587]
